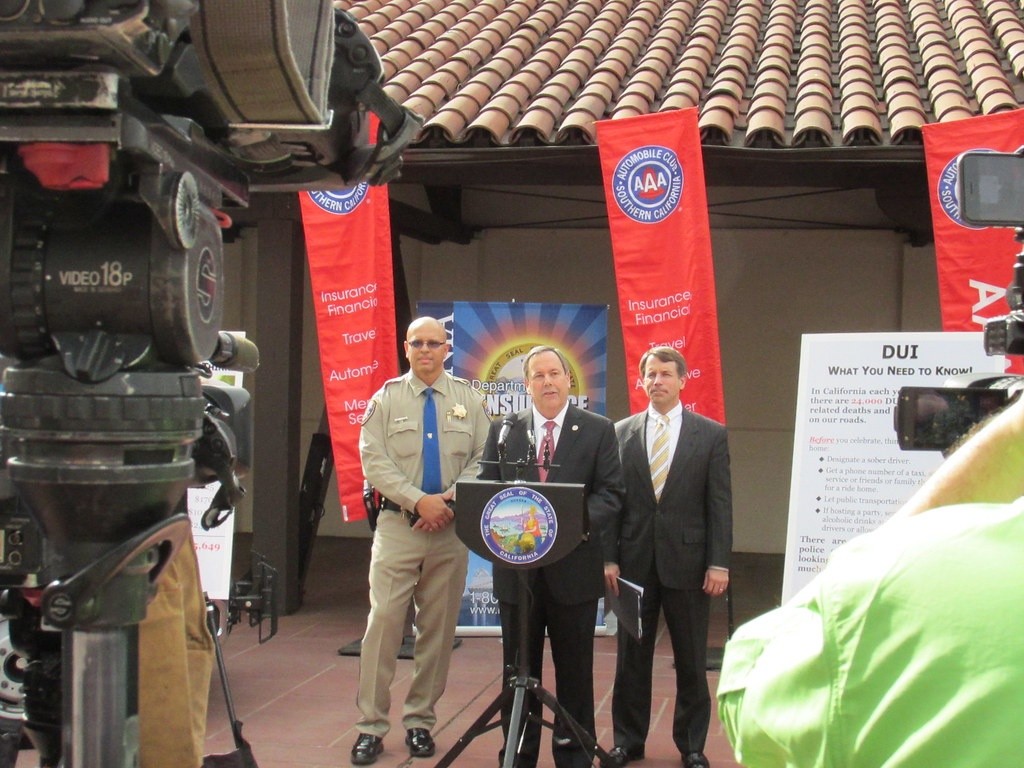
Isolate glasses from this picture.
[409,339,445,348]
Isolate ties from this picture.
[649,415,670,502]
[422,389,444,493]
[536,420,559,482]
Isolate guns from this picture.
[363,480,376,531]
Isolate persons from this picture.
[475,344,626,768]
[350,318,492,764]
[604,347,733,768]
[716,394,1024,768]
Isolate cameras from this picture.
[896,375,1024,459]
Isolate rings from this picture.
[720,589,723,591]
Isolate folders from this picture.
[607,577,644,646]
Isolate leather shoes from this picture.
[351,733,384,765]
[684,751,710,768]
[406,728,435,757]
[599,745,645,768]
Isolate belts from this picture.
[383,498,399,510]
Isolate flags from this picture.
[298,112,399,523]
[922,108,1024,376]
[594,106,726,425]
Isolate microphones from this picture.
[498,412,518,446]
[210,331,259,373]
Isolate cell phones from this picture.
[956,152,1024,230]
